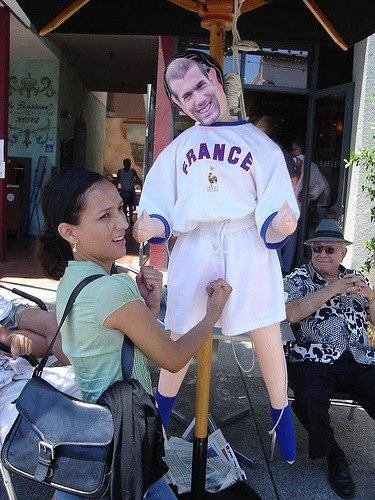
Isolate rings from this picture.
[353,282,355,286]
[357,285,362,292]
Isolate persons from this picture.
[113,159,142,227]
[283,218,375,499]
[0,293,86,447]
[263,138,330,276]
[131,50,301,470]
[36,169,232,500]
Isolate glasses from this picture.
[312,248,344,254]
[290,148,299,151]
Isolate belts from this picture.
[317,206,328,208]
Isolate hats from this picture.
[304,219,352,246]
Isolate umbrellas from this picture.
[17,0,374,100]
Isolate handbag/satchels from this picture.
[1,275,135,500]
